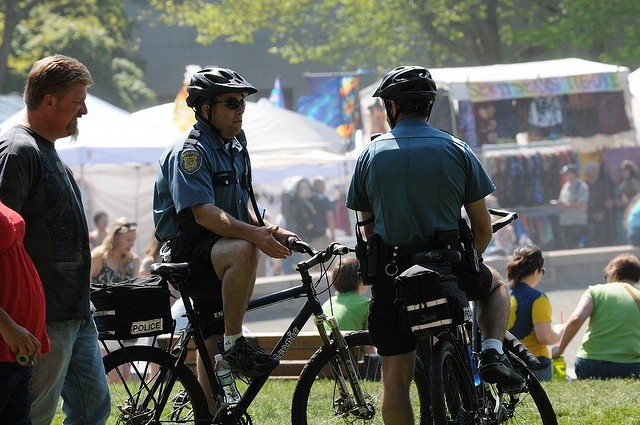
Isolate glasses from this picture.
[539,269,546,274]
[209,98,246,109]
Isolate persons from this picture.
[88,213,112,252]
[368,97,388,141]
[606,159,638,244]
[343,65,524,423]
[314,253,376,361]
[1,199,50,425]
[308,175,337,272]
[0,55,111,425]
[248,192,270,277]
[550,164,590,248]
[505,243,567,381]
[551,253,640,380]
[171,295,194,334]
[153,68,300,415]
[281,180,316,274]
[90,217,140,383]
[138,238,175,385]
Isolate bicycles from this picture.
[91,237,431,425]
[354,207,560,424]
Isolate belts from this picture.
[384,244,427,258]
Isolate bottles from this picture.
[551,356,566,382]
[215,352,241,407]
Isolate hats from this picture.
[558,162,579,176]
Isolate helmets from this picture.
[372,66,438,100]
[186,68,258,107]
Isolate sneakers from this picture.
[477,348,526,388]
[219,336,279,376]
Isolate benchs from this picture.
[154,330,364,383]
